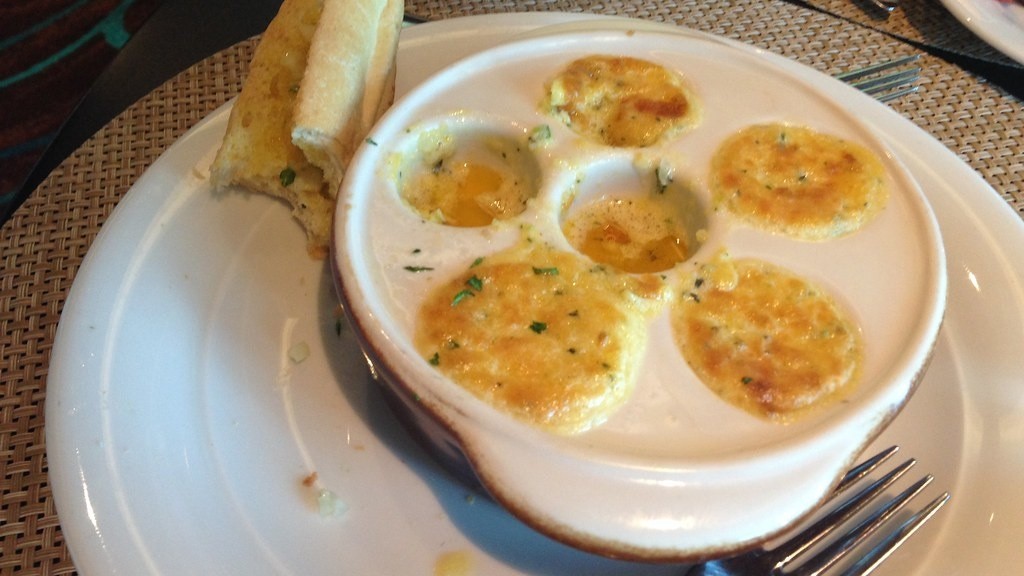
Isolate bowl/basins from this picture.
[334,30,947,563]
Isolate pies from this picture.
[210,0,405,258]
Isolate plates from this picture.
[944,0,1024,64]
[45,11,1024,576]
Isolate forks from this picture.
[685,445,951,576]
[833,54,923,102]
